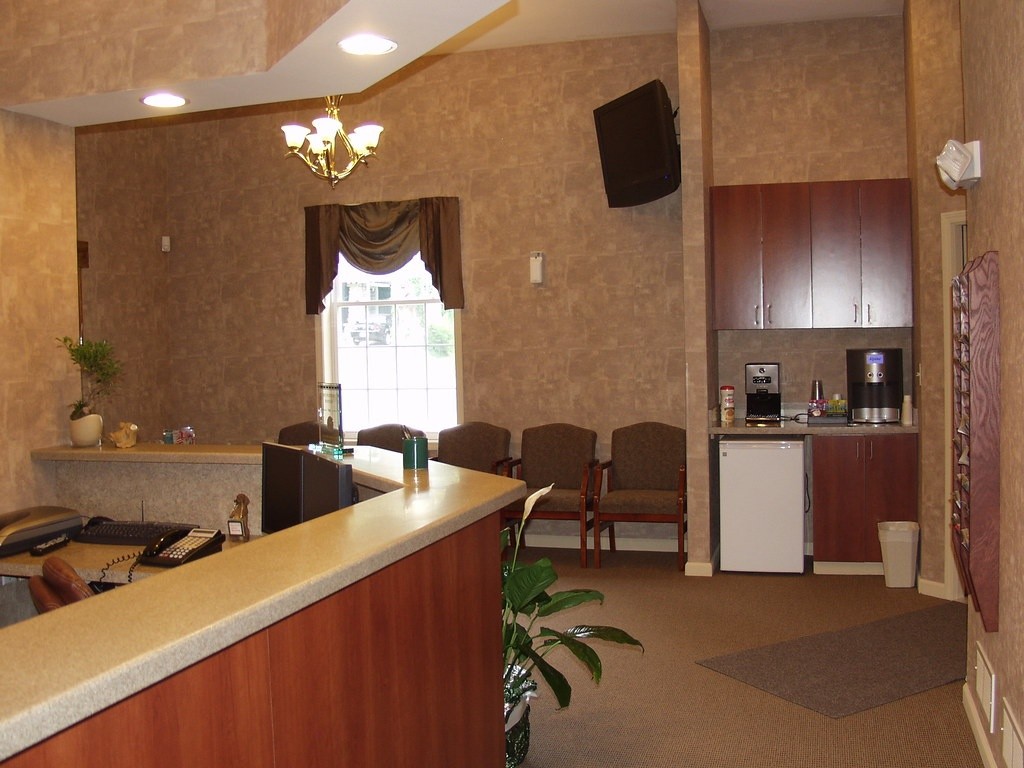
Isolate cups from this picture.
[811,380,824,400]
[403,436,428,470]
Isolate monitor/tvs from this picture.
[593,77,681,208]
[260,441,359,534]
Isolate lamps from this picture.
[936,139,982,192]
[281,94,385,190]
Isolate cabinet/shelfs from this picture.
[952,251,998,631]
[812,434,920,575]
[709,178,915,331]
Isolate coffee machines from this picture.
[744,362,782,422]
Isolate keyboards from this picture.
[75,521,200,546]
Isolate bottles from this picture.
[902,395,913,425]
[720,386,735,422]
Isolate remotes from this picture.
[29,537,67,557]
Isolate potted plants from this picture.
[499,483,646,768]
[56,337,125,448]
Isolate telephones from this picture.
[140,526,225,567]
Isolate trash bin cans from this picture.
[878,521,919,587]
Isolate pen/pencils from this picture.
[401,425,412,440]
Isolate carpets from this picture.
[694,601,968,719]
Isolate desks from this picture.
[0,537,264,585]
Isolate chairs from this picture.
[28,557,93,615]
[277,421,688,572]
